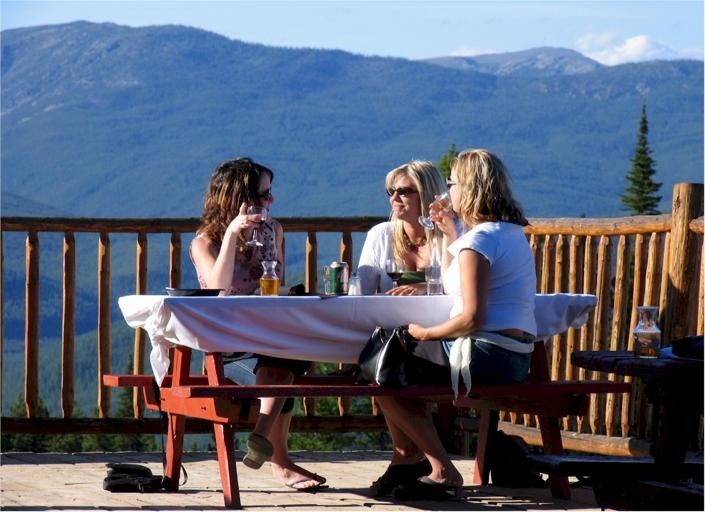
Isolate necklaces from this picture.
[402,227,428,252]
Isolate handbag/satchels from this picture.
[491,432,546,488]
[104,464,164,492]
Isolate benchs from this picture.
[172,379,633,398]
[103,372,362,389]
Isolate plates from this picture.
[165,287,225,296]
[661,347,704,361]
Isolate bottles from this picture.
[348,272,361,296]
[259,260,280,296]
[632,307,661,359]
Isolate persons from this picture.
[189,157,329,493]
[367,148,539,502]
[346,160,446,296]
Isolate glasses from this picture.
[387,187,416,196]
[255,187,272,200]
[446,177,456,187]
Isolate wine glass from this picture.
[385,258,403,288]
[418,190,452,230]
[244,205,267,247]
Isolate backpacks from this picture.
[348,326,417,386]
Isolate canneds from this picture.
[329,262,349,296]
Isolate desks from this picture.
[117,293,599,510]
[570,350,703,499]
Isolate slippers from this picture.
[393,477,462,502]
[243,433,273,469]
[370,462,430,496]
[282,471,326,488]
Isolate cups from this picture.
[425,268,444,295]
[322,264,330,295]
